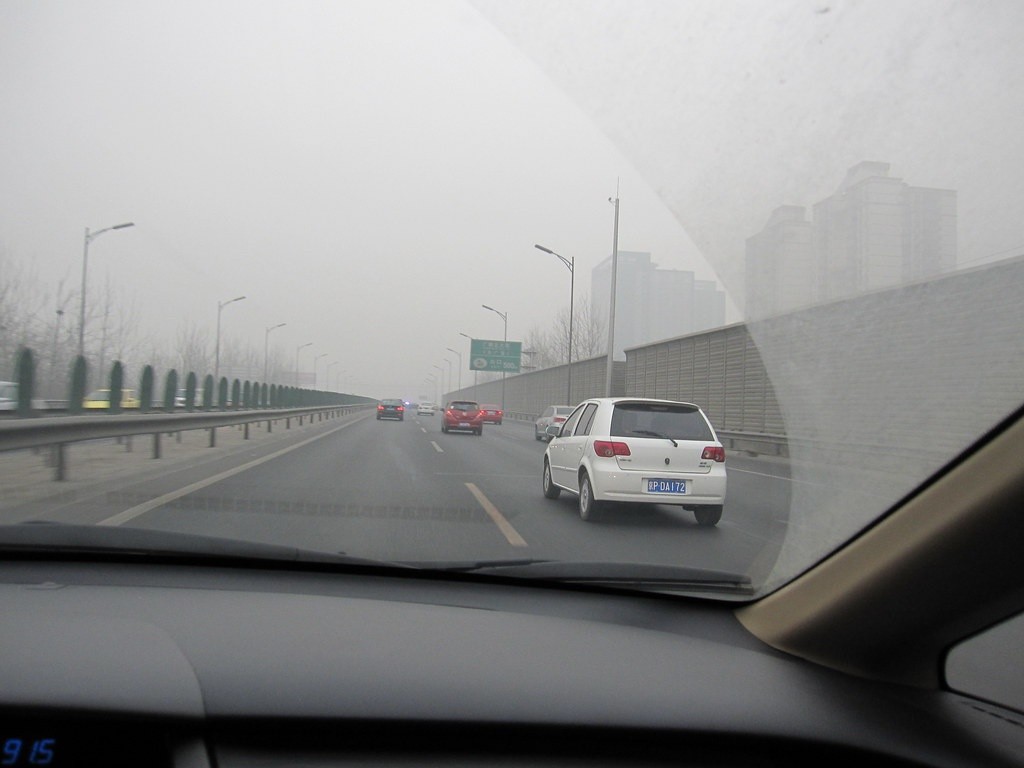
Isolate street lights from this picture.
[433,364,444,396]
[534,243,575,408]
[313,353,328,390]
[214,296,248,380]
[296,342,313,387]
[78,222,134,353]
[458,333,477,401]
[264,323,286,384]
[481,305,507,412]
[446,348,461,401]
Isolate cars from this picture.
[534,405,577,443]
[376,399,404,422]
[478,402,502,425]
[81,388,141,409]
[174,387,204,409]
[543,396,728,526]
[417,402,435,417]
[0,382,52,411]
[439,400,484,436]
